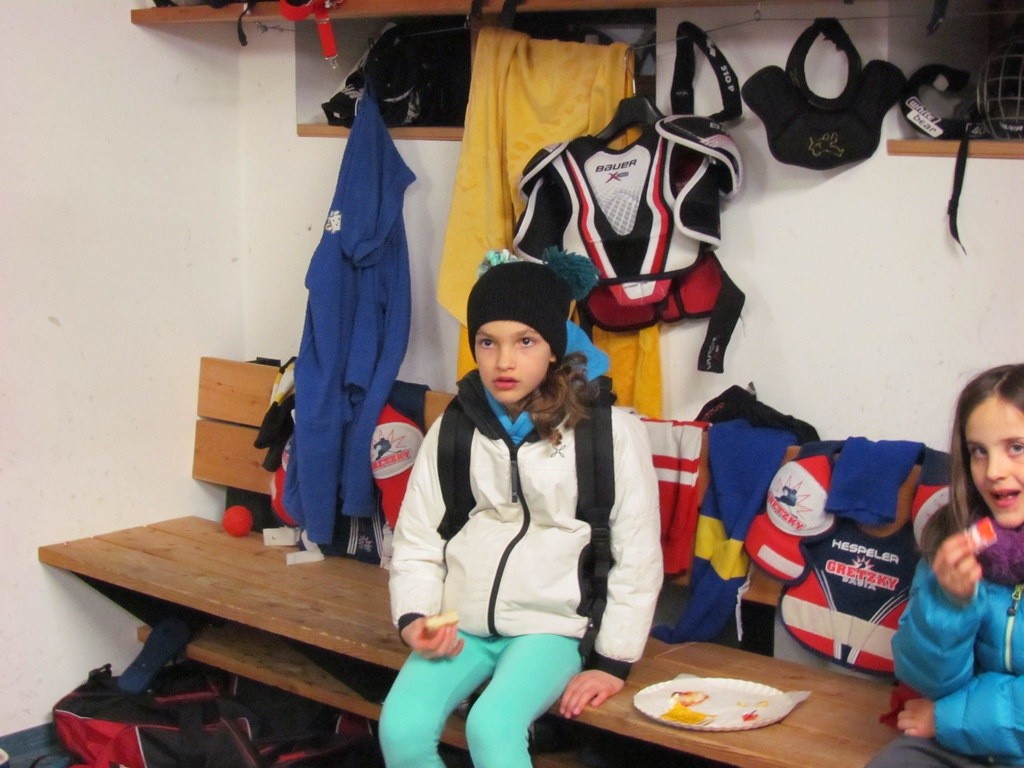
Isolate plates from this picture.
[633,675,794,732]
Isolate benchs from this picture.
[34,356,908,767]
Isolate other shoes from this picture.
[118,616,191,694]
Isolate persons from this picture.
[378,245,665,768]
[866,363,1024,768]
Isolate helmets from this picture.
[977,35,1024,143]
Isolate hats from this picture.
[467,253,597,365]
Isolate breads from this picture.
[425,611,459,633]
[966,515,997,553]
